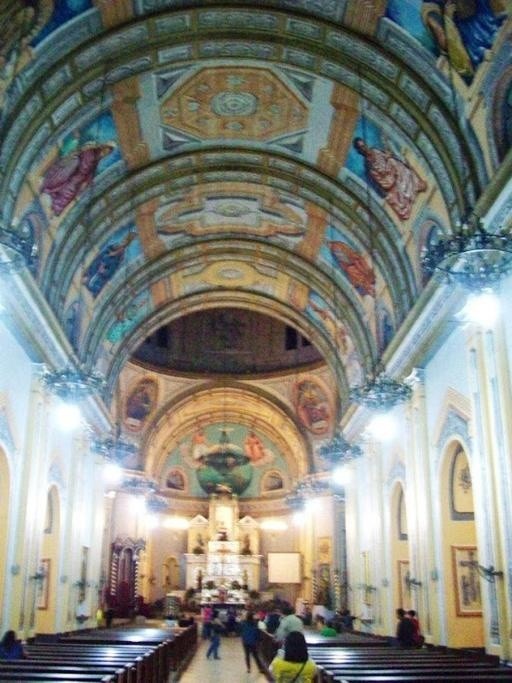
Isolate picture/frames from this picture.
[37,556,51,609]
[396,489,411,540]
[450,542,483,616]
[397,557,413,613]
[447,442,474,521]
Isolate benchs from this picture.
[2,618,198,682]
[248,618,512,682]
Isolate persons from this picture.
[300,380,329,431]
[324,235,378,298]
[103,609,112,629]
[178,592,353,683]
[0,630,28,659]
[141,600,163,619]
[396,608,413,649]
[419,2,512,88]
[83,231,137,298]
[1,1,54,89]
[308,300,349,354]
[408,610,421,646]
[107,298,148,346]
[129,379,156,420]
[353,137,426,221]
[194,426,266,475]
[40,140,115,215]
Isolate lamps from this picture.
[316,189,363,465]
[44,58,110,404]
[80,181,138,461]
[416,0,511,334]
[347,61,415,416]
